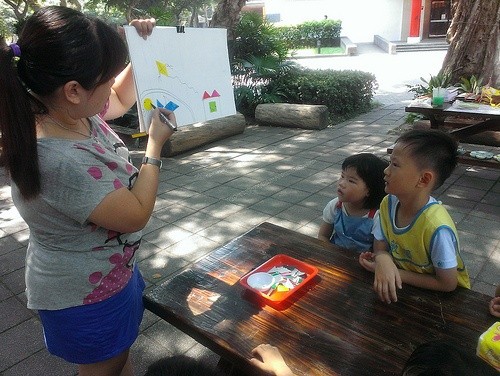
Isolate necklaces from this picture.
[47,113,99,142]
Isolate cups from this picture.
[433,87,444,106]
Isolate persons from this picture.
[212,343,295,376]
[359,129,471,304]
[318,153,391,254]
[0,6,177,376]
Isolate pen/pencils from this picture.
[151,103,177,131]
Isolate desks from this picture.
[396,96,500,142]
[141,221,500,376]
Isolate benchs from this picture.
[387,143,500,169]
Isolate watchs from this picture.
[142,156,163,169]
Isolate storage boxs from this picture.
[238,253,319,311]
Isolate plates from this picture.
[247,272,276,292]
[239,253,319,307]
